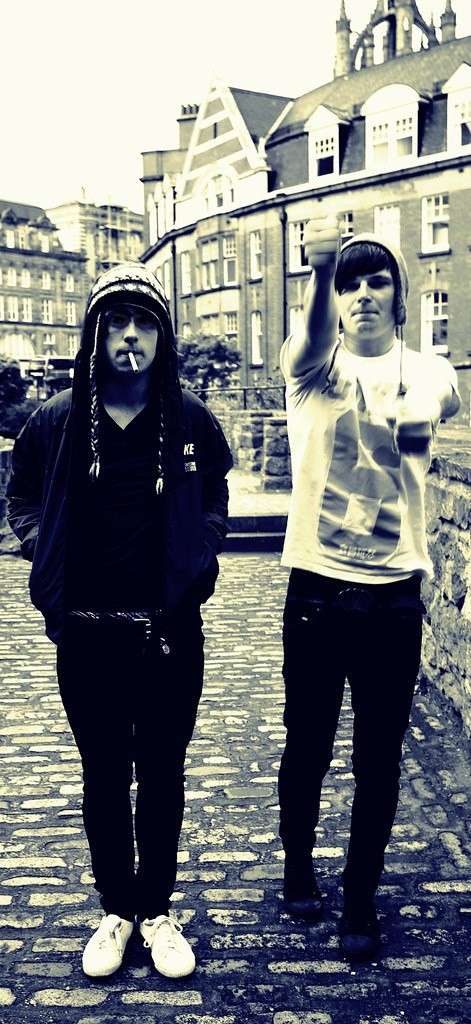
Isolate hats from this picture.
[87,290,171,494]
[322,231,409,397]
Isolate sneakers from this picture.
[82,914,136,983]
[138,914,196,982]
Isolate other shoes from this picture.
[338,864,382,959]
[282,854,324,919]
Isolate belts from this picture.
[308,582,427,618]
[66,608,171,654]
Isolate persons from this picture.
[4,264,234,980]
[277,215,461,959]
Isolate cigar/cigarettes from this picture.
[128,352,139,373]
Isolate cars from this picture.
[20,356,75,390]
